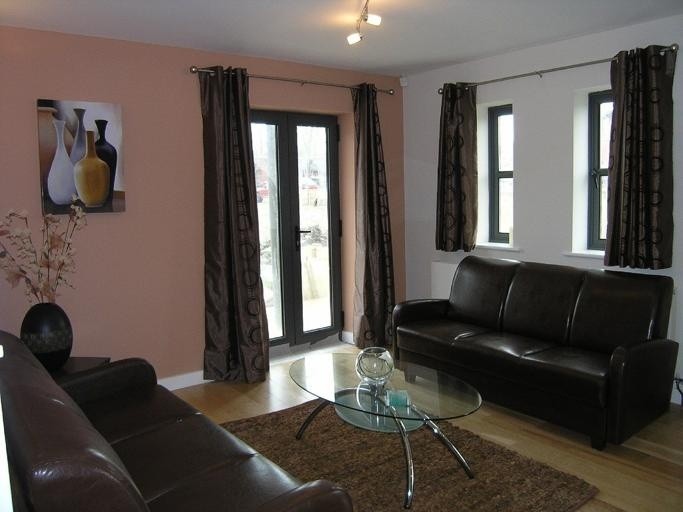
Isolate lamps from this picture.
[346,0,381,47]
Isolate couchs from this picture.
[0,329,353,510]
[390,256,679,452]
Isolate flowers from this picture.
[0,205,86,305]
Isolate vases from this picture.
[18,303,71,372]
[46,109,117,211]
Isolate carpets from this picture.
[217,383,598,510]
